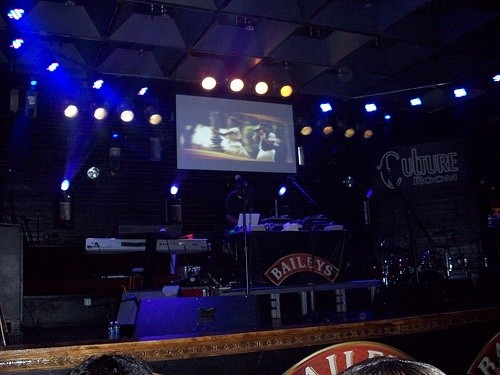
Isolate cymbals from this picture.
[431,228,457,236]
[376,247,411,254]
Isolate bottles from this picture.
[108,322,113,339]
[113,321,119,340]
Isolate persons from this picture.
[68,352,154,375]
[224,174,269,287]
[340,356,446,375]
[191,110,279,162]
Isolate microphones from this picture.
[235,174,248,186]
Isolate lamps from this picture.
[59,98,80,122]
[197,69,218,92]
[224,72,247,96]
[143,105,162,125]
[337,118,356,139]
[55,175,72,201]
[315,118,335,137]
[117,103,135,124]
[89,101,112,125]
[296,117,313,136]
[167,180,179,203]
[85,69,106,92]
[274,80,295,99]
[358,119,375,142]
[251,76,272,97]
[271,176,288,197]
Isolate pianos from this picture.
[86,225,212,291]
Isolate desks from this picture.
[219,279,382,319]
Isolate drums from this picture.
[419,249,448,271]
[445,253,487,274]
[379,255,412,278]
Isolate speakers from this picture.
[132,292,263,340]
[0,223,26,322]
[371,271,451,319]
